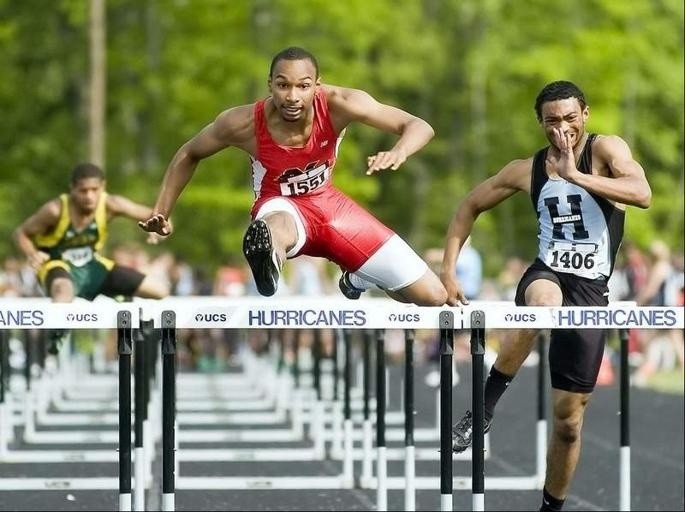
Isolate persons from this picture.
[138,47,448,308]
[439,80,653,512]
[11,162,173,355]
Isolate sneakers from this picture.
[448,410,495,453]
[242,219,281,297]
[47,329,71,356]
[338,270,365,301]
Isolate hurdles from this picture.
[0,296,141,512]
[463,305,684,511]
[153,297,462,512]
[0,294,631,512]
